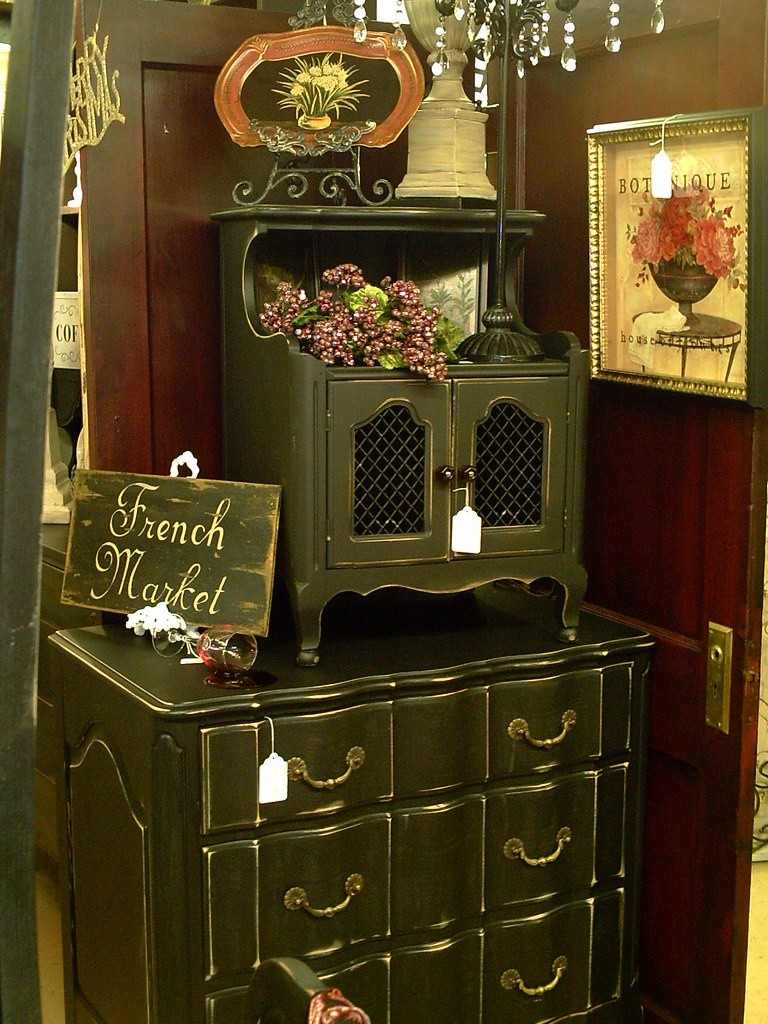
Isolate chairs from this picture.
[242,957,372,1023]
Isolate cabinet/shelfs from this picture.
[49,602,657,1023]
[207,205,592,667]
[34,525,73,875]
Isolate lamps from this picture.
[345,0,668,362]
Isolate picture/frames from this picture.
[213,24,426,149]
[584,106,768,408]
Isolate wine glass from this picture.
[152,613,258,674]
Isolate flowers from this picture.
[626,179,745,293]
[262,262,466,383]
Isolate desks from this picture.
[631,312,743,382]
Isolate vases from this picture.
[645,260,720,326]
